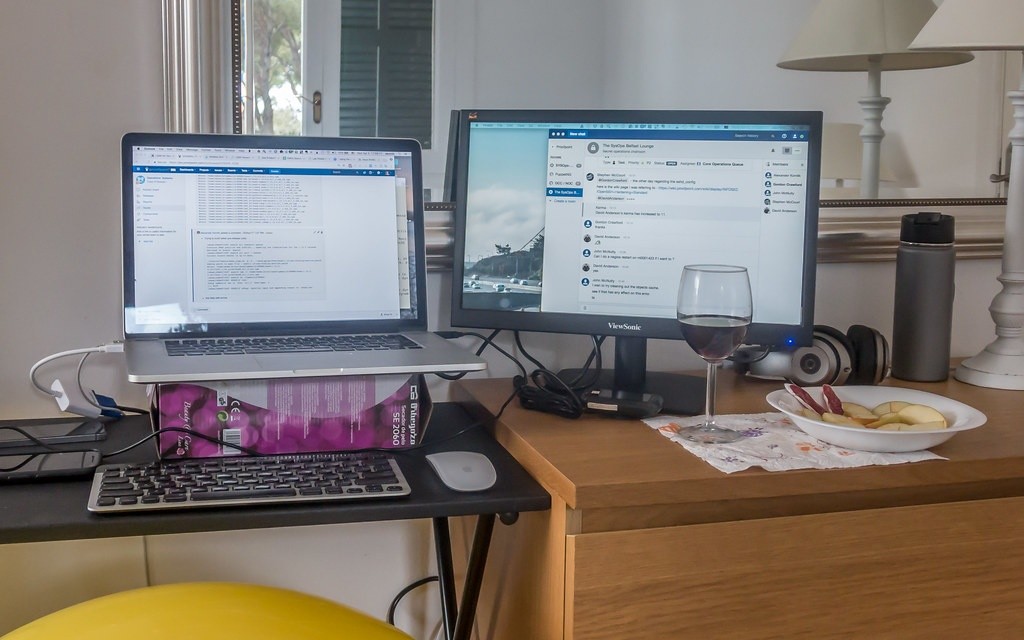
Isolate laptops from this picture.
[119,132,487,385]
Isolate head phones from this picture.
[745,323,889,387]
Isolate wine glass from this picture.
[677,264,753,444]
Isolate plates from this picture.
[766,384,986,453]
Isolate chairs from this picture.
[0,581,413,640]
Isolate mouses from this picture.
[425,450,497,491]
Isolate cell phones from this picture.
[0,451,101,483]
[0,422,107,448]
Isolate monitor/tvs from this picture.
[451,111,824,418]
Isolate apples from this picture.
[802,401,948,432]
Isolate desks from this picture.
[450,355,1024,640]
[0,406,552,640]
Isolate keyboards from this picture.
[88,453,411,514]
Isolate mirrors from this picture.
[162,0,1024,264]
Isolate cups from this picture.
[891,211,956,382]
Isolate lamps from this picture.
[907,0,1024,392]
[820,123,891,188]
[775,0,974,200]
